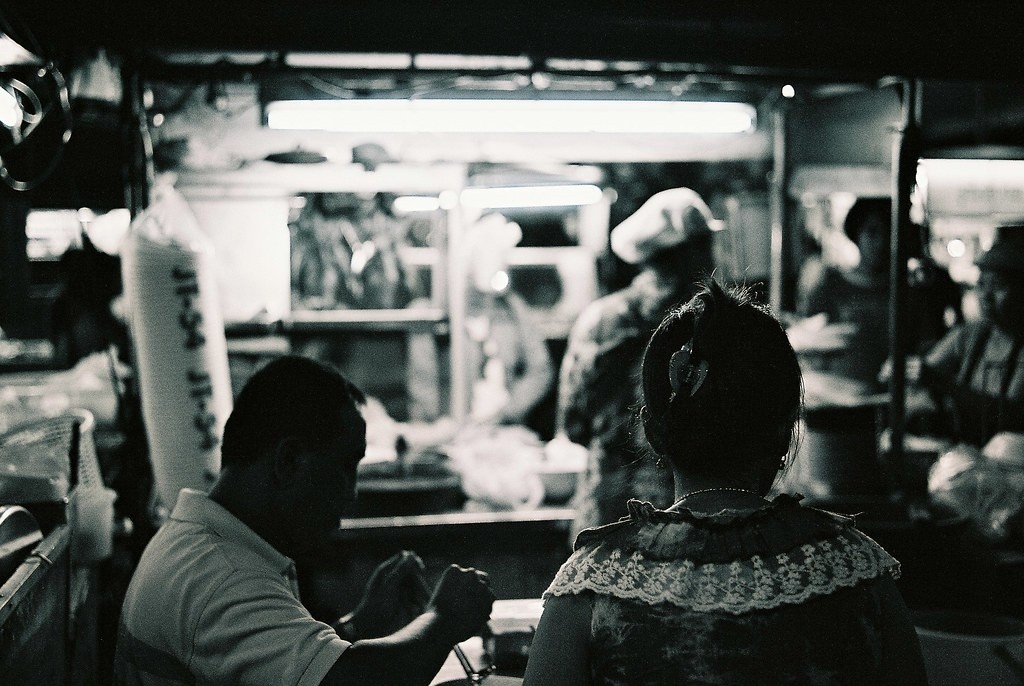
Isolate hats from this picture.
[975,226,1023,271]
[611,186,724,261]
[841,195,890,232]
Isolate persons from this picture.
[920,218,1024,449]
[524,265,928,686]
[805,196,945,380]
[109,354,495,686]
[565,188,725,550]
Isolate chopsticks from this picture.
[400,549,480,685]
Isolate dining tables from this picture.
[427,598,545,684]
